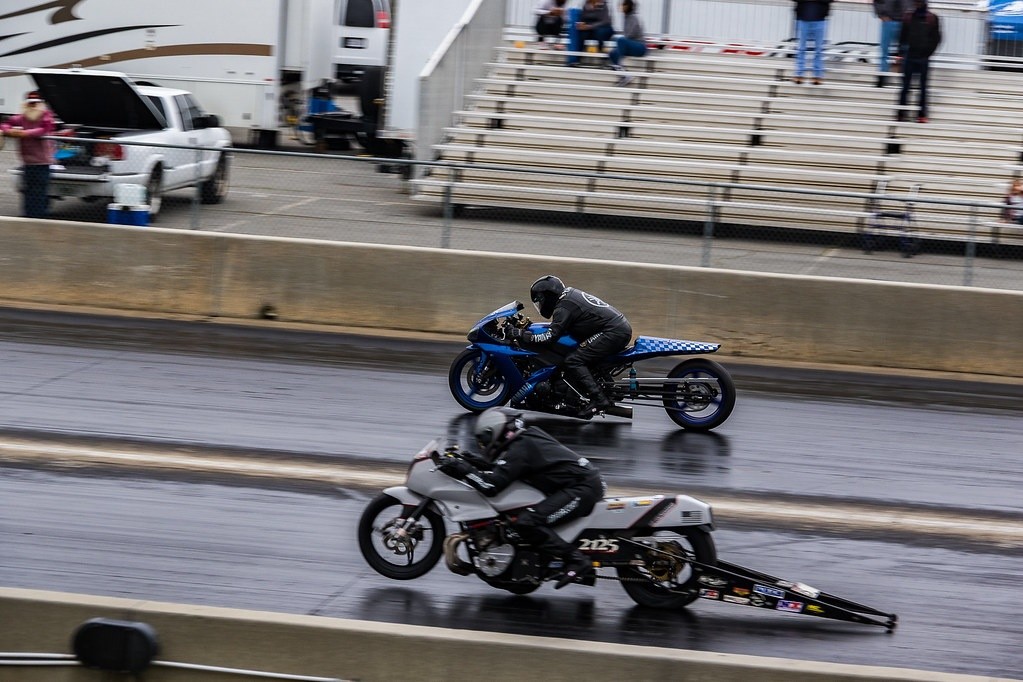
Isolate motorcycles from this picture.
[448,300,737,431]
[357,438,899,631]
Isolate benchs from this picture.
[410,42,1023,248]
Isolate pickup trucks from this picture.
[7,67,232,223]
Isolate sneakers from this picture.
[554,553,593,590]
[577,392,612,418]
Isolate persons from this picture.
[608,0,647,88]
[790,0,834,85]
[571,0,613,64]
[441,407,607,591]
[0,90,56,219]
[531,0,567,42]
[872,0,913,88]
[509,274,633,417]
[894,0,943,123]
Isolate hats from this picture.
[25,90,44,103]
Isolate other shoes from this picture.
[812,78,822,85]
[897,113,910,122]
[616,74,634,89]
[875,79,885,88]
[917,115,929,123]
[604,63,624,72]
[794,77,803,84]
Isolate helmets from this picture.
[473,407,524,456]
[530,275,565,320]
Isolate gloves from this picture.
[441,458,474,482]
[506,328,532,344]
